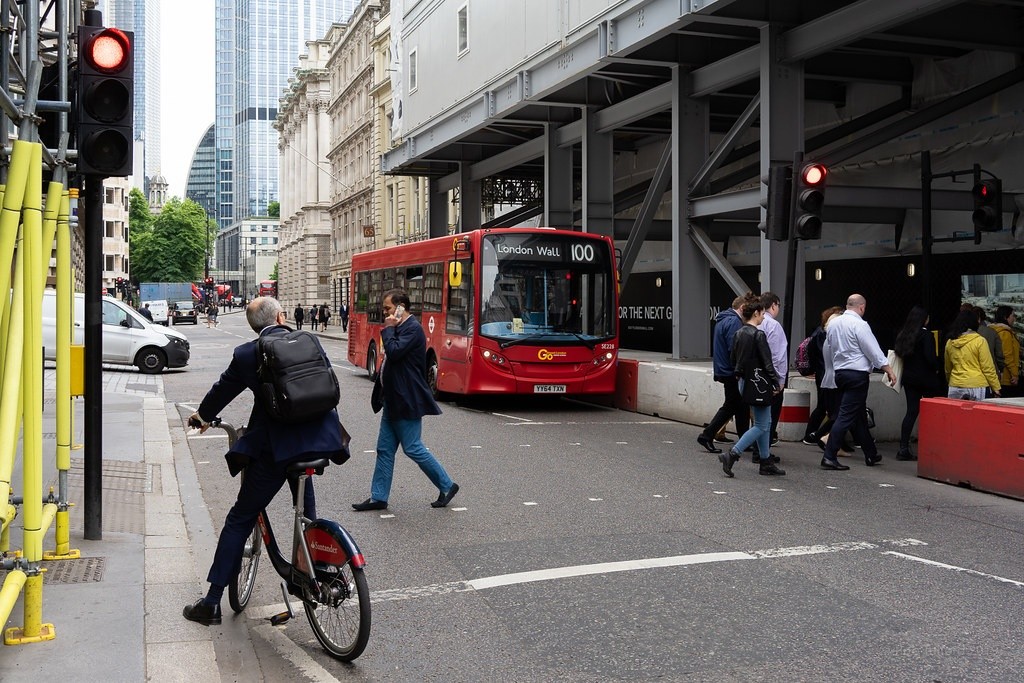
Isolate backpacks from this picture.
[795,326,821,377]
[253,324,342,421]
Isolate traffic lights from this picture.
[118,277,123,289]
[208,277,214,289]
[205,278,209,289]
[972,178,1003,233]
[793,162,827,240]
[758,165,793,242]
[76,25,134,178]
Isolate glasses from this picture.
[775,303,781,308]
[279,310,288,319]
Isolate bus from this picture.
[346,226,624,402]
[260,280,278,299]
[214,284,232,306]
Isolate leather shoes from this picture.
[183,598,221,625]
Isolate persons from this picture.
[294,303,304,330]
[309,302,331,332]
[352,290,459,510]
[547,291,560,327]
[803,294,897,470]
[206,303,220,328]
[897,303,1019,461]
[139,304,153,321]
[697,290,788,476]
[183,296,351,625]
[339,300,349,333]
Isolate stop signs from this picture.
[102,288,107,295]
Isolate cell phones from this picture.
[393,305,405,320]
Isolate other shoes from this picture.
[895,442,918,461]
[430,483,460,507]
[810,431,820,442]
[352,498,388,509]
[817,440,827,451]
[759,458,786,475]
[770,439,779,447]
[718,448,736,477]
[820,456,850,471]
[713,437,734,444]
[751,454,780,463]
[803,436,819,446]
[836,452,851,457]
[697,434,722,453]
[865,453,883,465]
[853,437,876,449]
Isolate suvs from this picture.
[171,301,199,325]
[9,288,191,374]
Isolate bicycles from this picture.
[186,416,371,661]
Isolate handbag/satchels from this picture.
[743,330,775,407]
[881,348,906,393]
[866,408,875,429]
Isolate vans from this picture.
[140,299,170,326]
